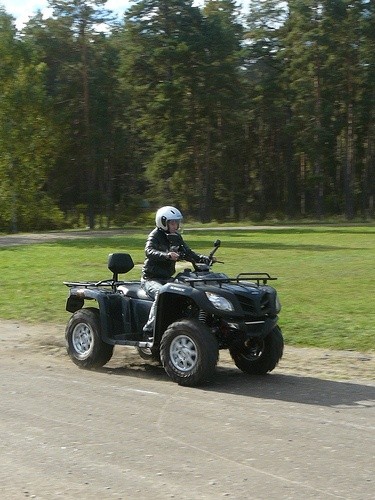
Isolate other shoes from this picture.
[144,331,153,342]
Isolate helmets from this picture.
[156,206,182,231]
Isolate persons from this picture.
[140,207,216,333]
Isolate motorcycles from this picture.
[63,238,284,388]
